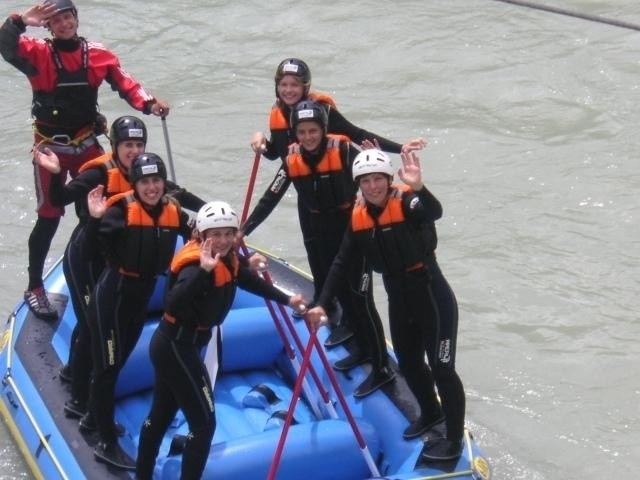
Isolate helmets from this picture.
[196,200,240,233]
[129,152,167,186]
[289,99,329,135]
[275,57,311,99]
[40,0,78,29]
[351,148,394,182]
[109,115,147,156]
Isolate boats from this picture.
[0,233,493,480]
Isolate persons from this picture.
[301,147,466,467]
[81,151,271,472]
[233,100,397,402]
[250,81,428,320]
[27,115,209,424]
[1,1,169,323]
[131,200,310,479]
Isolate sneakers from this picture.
[57,361,126,438]
[420,436,463,462]
[401,406,447,440]
[92,437,140,471]
[290,298,373,373]
[23,285,59,322]
[350,364,397,399]
